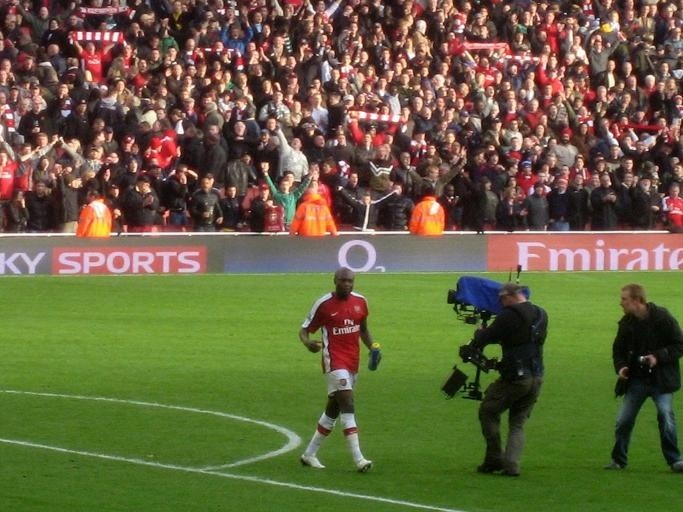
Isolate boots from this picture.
[477,431,504,473]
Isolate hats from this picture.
[369,95,382,104]
[496,282,522,304]
[520,160,532,168]
[258,183,269,190]
[561,128,572,137]
[343,95,353,101]
[460,111,469,118]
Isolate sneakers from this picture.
[356,459,371,471]
[603,463,623,470]
[300,453,325,468]
[670,460,683,470]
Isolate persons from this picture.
[298,267,382,474]
[602,283,683,473]
[474,281,549,477]
